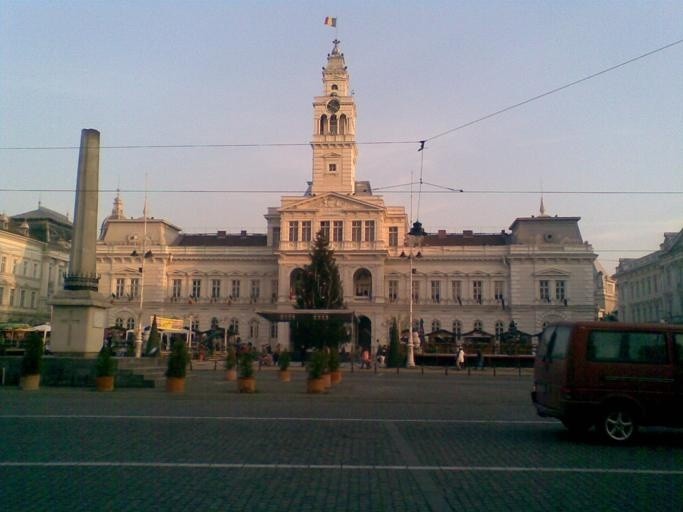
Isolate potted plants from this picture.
[19,331,43,390]
[165,337,187,395]
[306,337,342,394]
[276,347,291,383]
[94,346,117,392]
[225,345,261,393]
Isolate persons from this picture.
[456,345,464,370]
[271,342,280,367]
[358,346,372,370]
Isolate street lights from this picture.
[395,247,424,371]
[185,315,192,354]
[130,247,155,360]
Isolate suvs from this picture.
[527,318,682,447]
